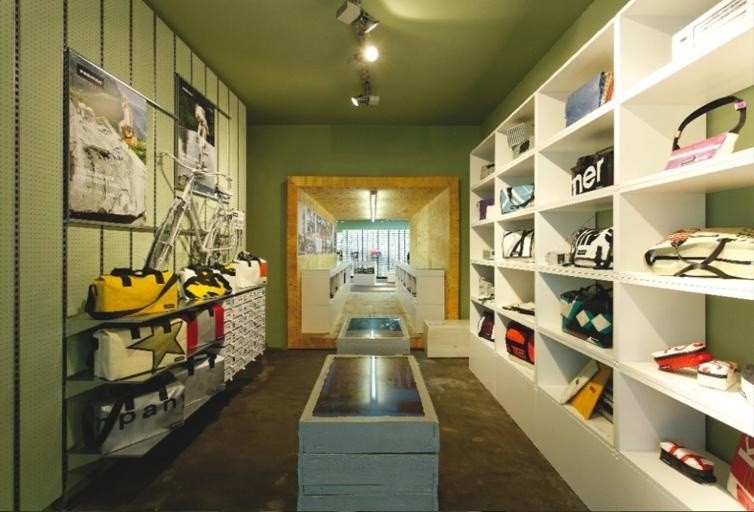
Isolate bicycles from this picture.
[144,151,246,272]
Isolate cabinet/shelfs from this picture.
[394,257,445,332]
[69,283,267,462]
[469,2,754,511]
[301,262,350,335]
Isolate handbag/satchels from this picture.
[84,265,181,321]
[82,370,186,454]
[641,226,754,280]
[173,302,226,348]
[659,440,717,484]
[725,432,754,511]
[559,283,613,350]
[168,350,226,409]
[479,69,614,179]
[80,316,188,383]
[662,95,745,171]
[476,182,534,364]
[697,359,742,391]
[559,226,614,272]
[652,340,714,372]
[567,143,616,197]
[231,251,268,289]
[179,260,238,301]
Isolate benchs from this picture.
[295,316,440,511]
[422,319,469,359]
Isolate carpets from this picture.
[328,293,416,338]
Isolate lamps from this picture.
[351,94,381,108]
[355,11,379,34]
